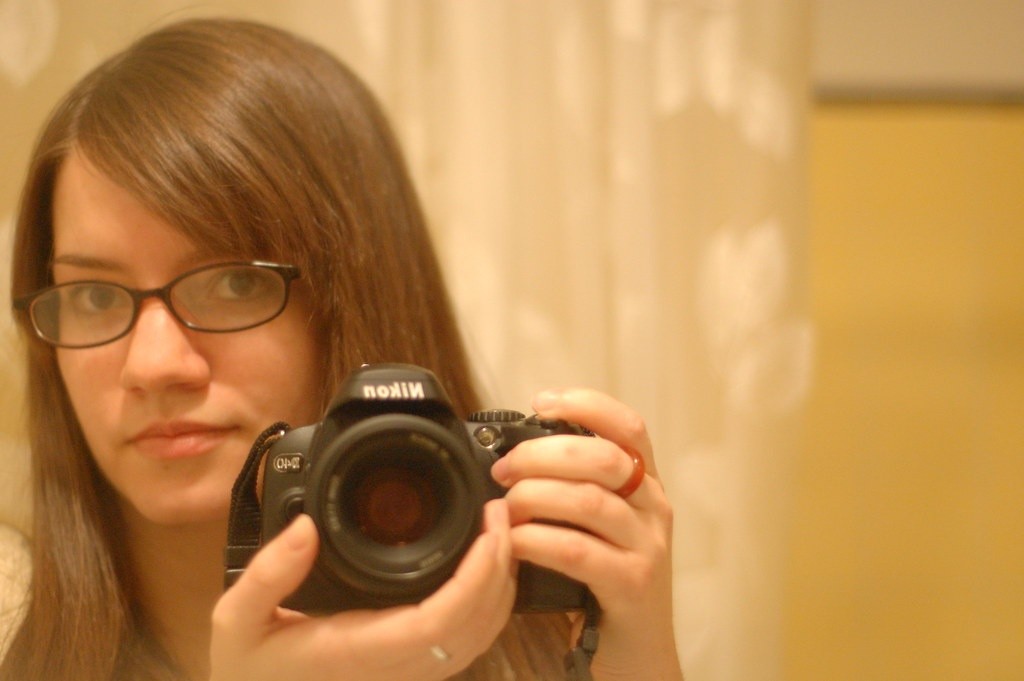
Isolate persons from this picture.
[0,18,685,681]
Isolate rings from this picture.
[611,443,648,501]
[427,645,453,666]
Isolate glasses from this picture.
[13,260,301,349]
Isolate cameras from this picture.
[258,363,590,614]
